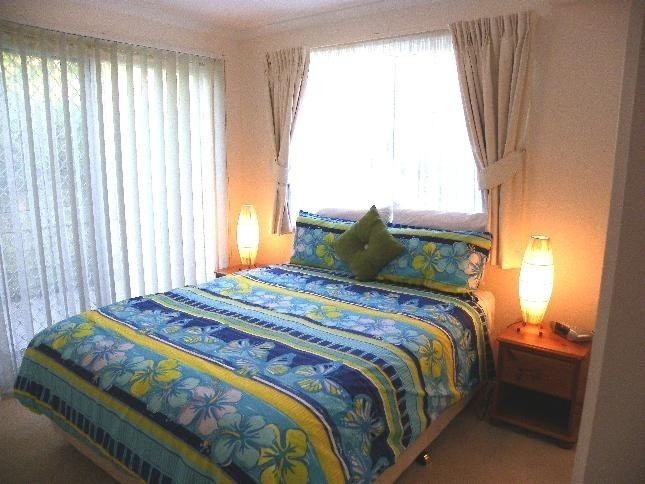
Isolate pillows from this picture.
[289,204,495,299]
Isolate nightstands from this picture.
[491,320,591,450]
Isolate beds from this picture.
[17,265,496,484]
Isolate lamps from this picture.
[512,232,554,338]
[236,206,262,270]
